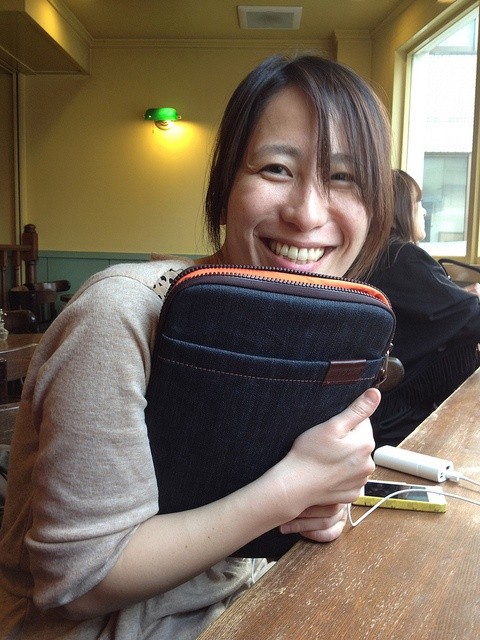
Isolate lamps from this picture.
[144,107,182,131]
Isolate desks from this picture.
[193,367,480,640]
[7,333,45,349]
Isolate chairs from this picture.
[0,310,38,334]
[10,280,70,333]
[0,343,38,445]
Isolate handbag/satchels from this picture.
[143,267,396,560]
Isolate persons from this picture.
[372,167,479,447]
[3,54,394,640]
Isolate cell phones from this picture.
[351,479,448,513]
